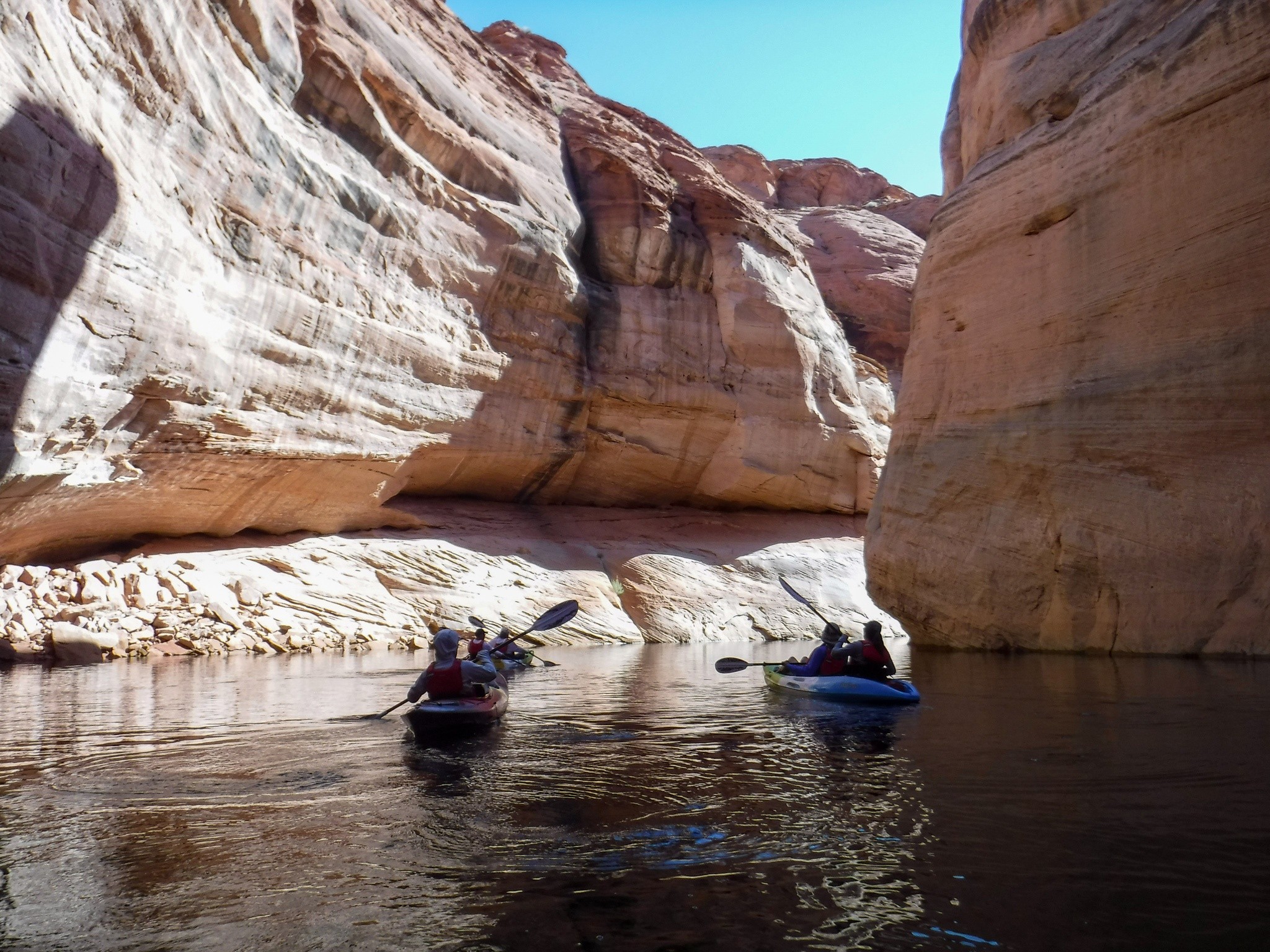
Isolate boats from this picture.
[472,648,535,672]
[762,660,921,705]
[398,672,509,736]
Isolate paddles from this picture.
[363,598,579,721]
[502,655,537,668]
[468,615,556,666]
[778,576,850,645]
[715,657,808,674]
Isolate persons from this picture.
[778,620,896,683]
[407,626,529,704]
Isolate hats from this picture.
[862,620,881,632]
[825,622,842,635]
[475,629,488,637]
[500,627,510,634]
